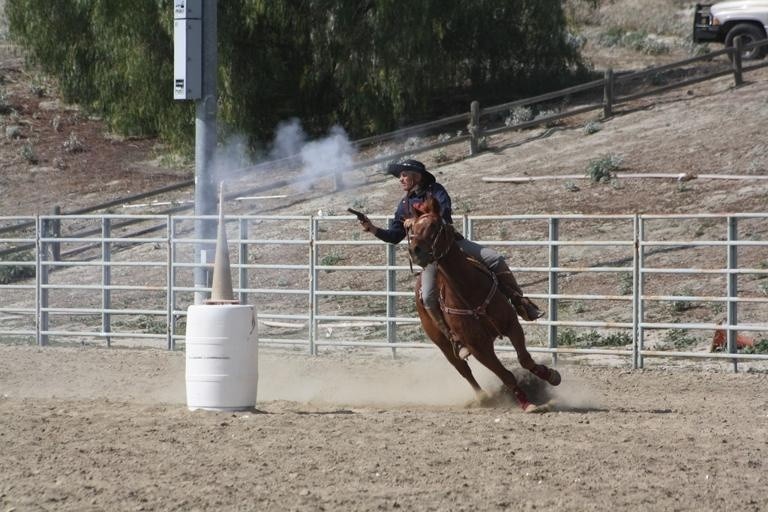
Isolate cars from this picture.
[692,1,767,62]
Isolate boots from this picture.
[496,263,545,320]
[425,305,470,360]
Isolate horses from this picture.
[400,196,562,413]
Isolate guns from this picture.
[347,208,365,224]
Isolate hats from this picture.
[387,160,436,185]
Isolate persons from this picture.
[358,159,545,361]
